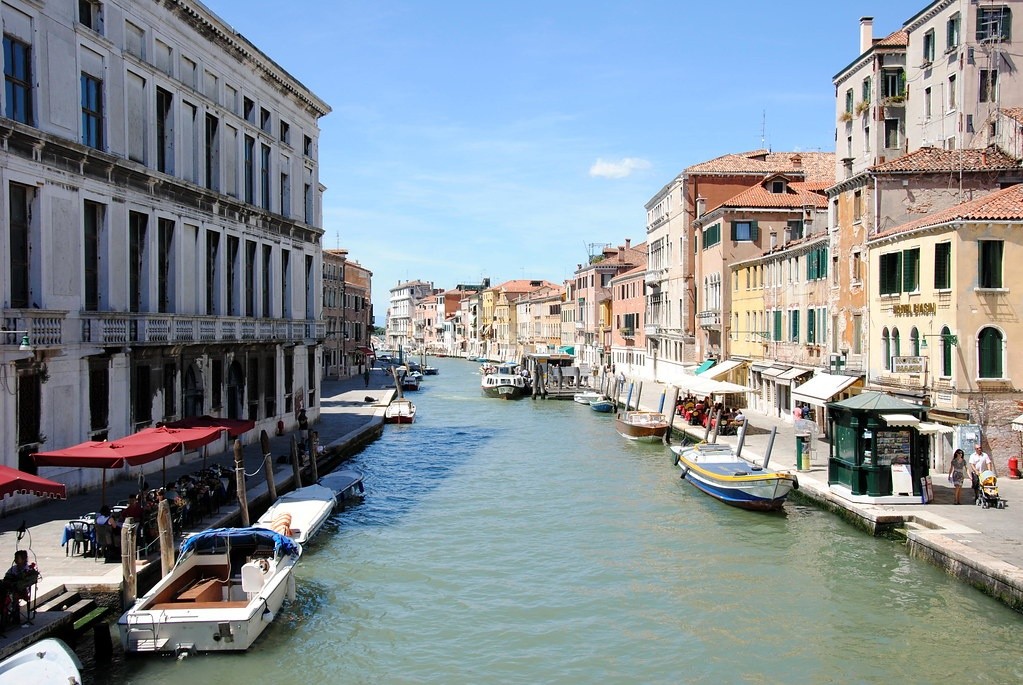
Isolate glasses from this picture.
[957,452,962,454]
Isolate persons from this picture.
[479,360,497,375]
[363,369,370,387]
[793,404,811,424]
[676,393,746,446]
[2,550,32,625]
[371,358,375,368]
[297,409,320,467]
[95,470,226,556]
[590,361,627,394]
[512,364,531,382]
[948,444,992,506]
[547,362,583,387]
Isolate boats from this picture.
[481,362,528,401]
[315,470,363,501]
[613,410,669,444]
[574,389,606,404]
[669,437,798,513]
[248,484,337,548]
[119,527,302,656]
[588,397,615,412]
[0,636,84,685]
[379,355,439,390]
[385,397,416,424]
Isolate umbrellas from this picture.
[28,440,173,507]
[110,425,221,486]
[155,415,255,468]
[657,372,761,411]
[0,464,67,501]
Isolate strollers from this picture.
[976,471,1004,509]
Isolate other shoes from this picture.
[953,500,961,505]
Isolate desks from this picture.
[62,523,124,557]
[675,402,736,432]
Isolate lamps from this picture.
[0,330,32,351]
[729,330,770,340]
[921,333,957,349]
[325,330,349,341]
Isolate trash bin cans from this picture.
[796,434,811,473]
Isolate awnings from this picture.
[694,359,745,379]
[1011,413,1023,432]
[878,413,968,435]
[792,372,859,408]
[761,363,814,386]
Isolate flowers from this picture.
[3,562,38,586]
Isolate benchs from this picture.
[176,579,222,603]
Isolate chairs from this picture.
[65,468,234,560]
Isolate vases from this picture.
[3,571,38,593]
